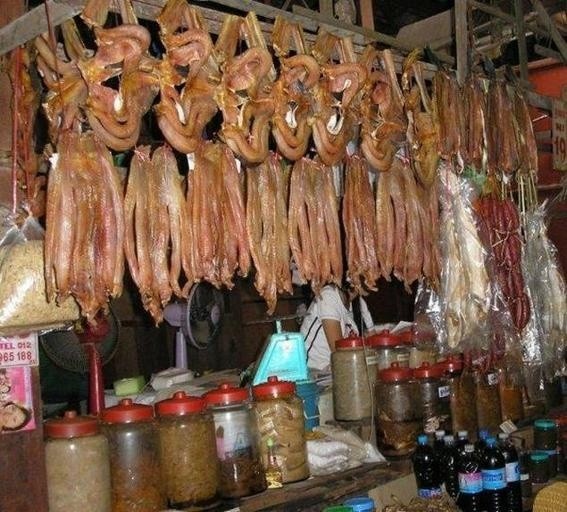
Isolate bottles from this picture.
[155,390,219,508]
[458,444,482,512]
[400,326,435,367]
[251,374,310,484]
[498,366,523,423]
[203,382,267,500]
[414,361,452,434]
[330,331,377,422]
[368,329,411,369]
[476,430,489,456]
[481,438,508,512]
[457,430,470,451]
[443,435,459,498]
[434,430,446,459]
[412,435,442,498]
[519,451,532,498]
[444,354,476,443]
[44,409,109,512]
[497,433,523,512]
[103,398,167,511]
[474,352,502,433]
[374,360,421,458]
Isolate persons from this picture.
[0,402,32,433]
[298,267,373,375]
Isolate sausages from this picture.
[475,190,531,335]
